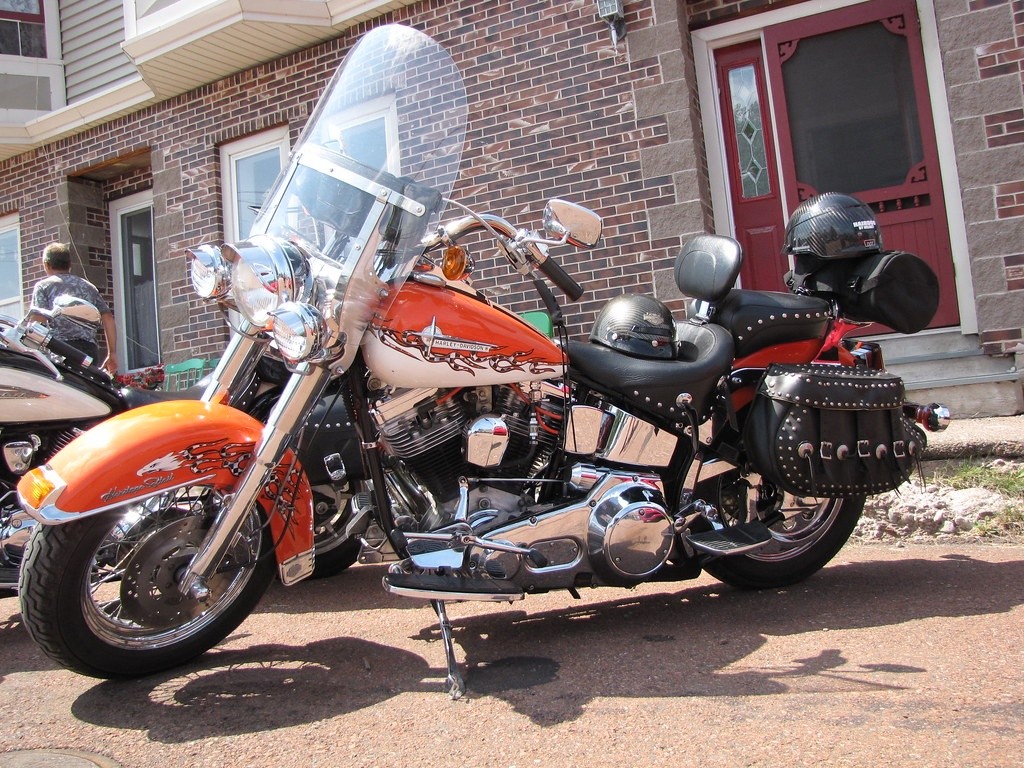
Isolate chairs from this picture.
[165,357,207,392]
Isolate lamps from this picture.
[597,0,627,55]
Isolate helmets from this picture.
[588,290,681,359]
[780,191,883,259]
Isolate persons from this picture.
[29,242,117,374]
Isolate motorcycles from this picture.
[0,313,386,593]
[13,21,950,702]
